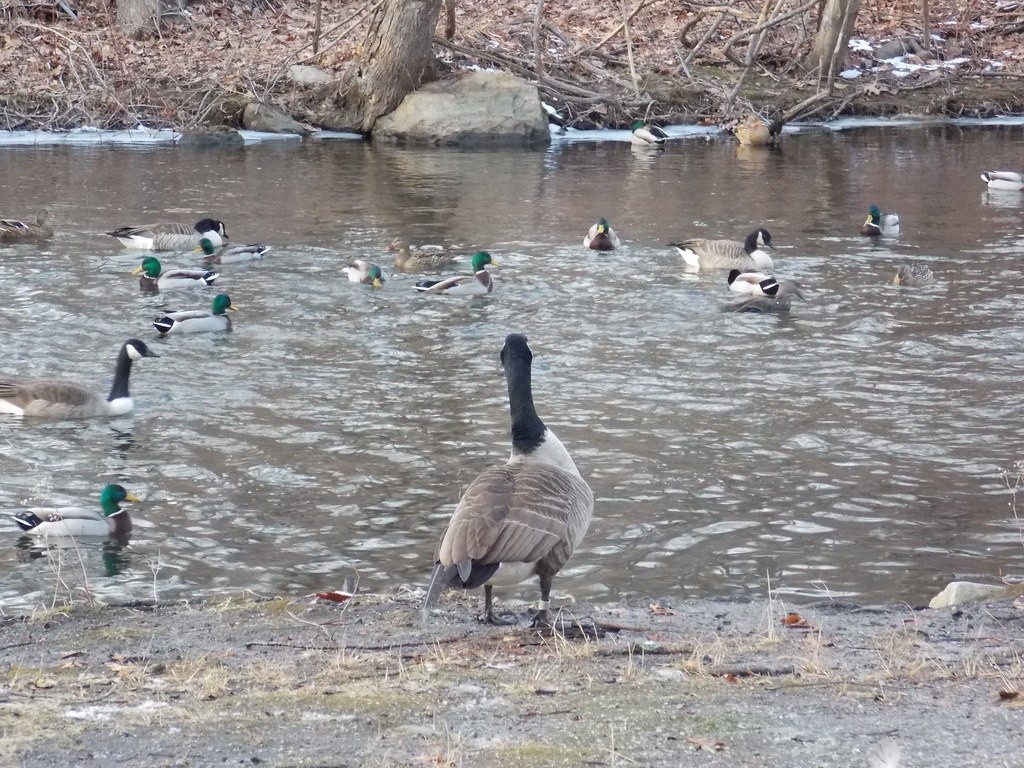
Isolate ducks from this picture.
[341,258,385,288]
[412,250,502,295]
[132,256,222,292]
[861,204,899,238]
[9,483,144,537]
[981,170,1024,191]
[582,217,620,251]
[629,120,674,146]
[152,294,239,334]
[192,238,273,265]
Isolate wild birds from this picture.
[0,210,55,239]
[894,264,935,287]
[106,219,231,252]
[420,333,594,630]
[715,279,807,314]
[731,119,784,146]
[0,338,160,418]
[727,267,778,296]
[385,238,464,270]
[667,228,779,270]
